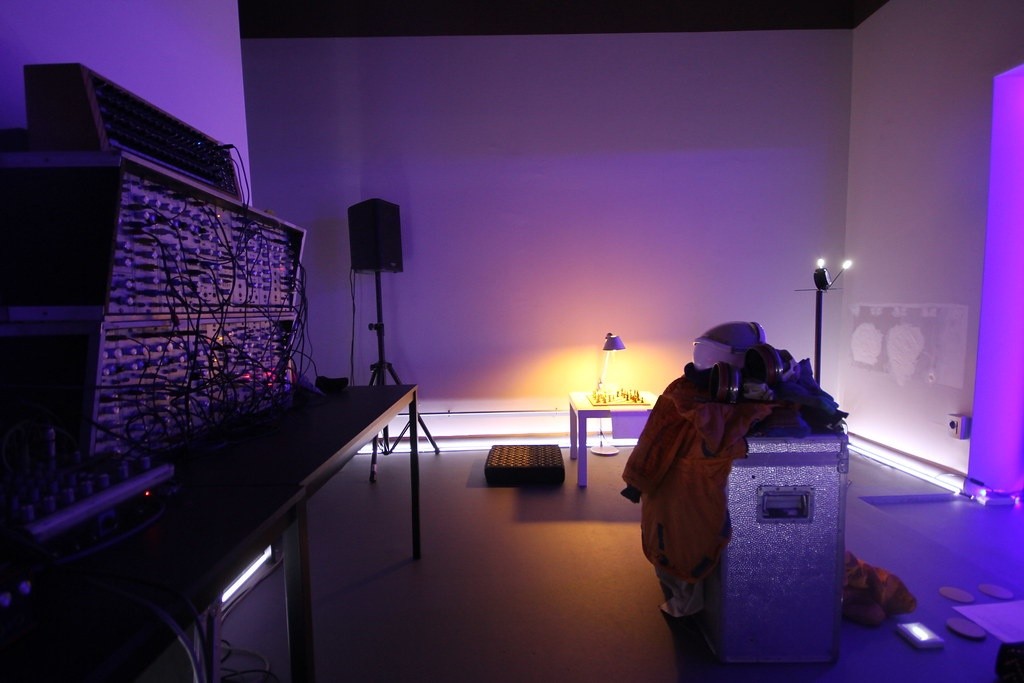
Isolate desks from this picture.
[568,389,658,488]
[0,382,420,683]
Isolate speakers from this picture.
[348,198,403,275]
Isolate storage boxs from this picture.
[702,435,850,662]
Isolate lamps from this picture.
[813,258,852,388]
[598,332,627,391]
[898,620,944,650]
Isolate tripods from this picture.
[350,275,438,483]
